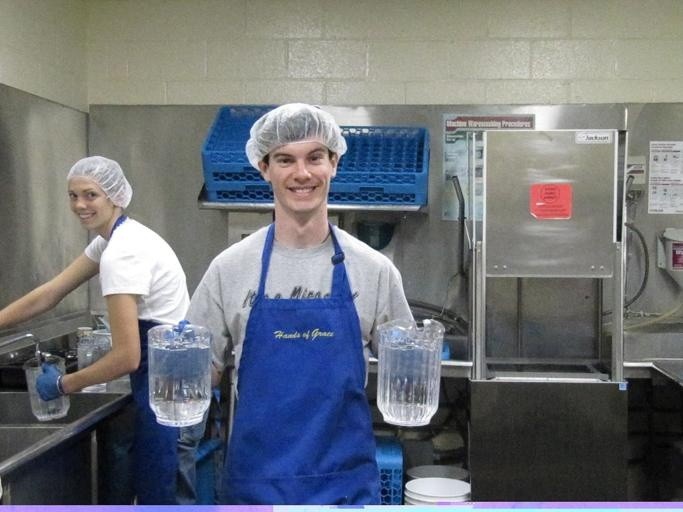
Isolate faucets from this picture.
[0,333,40,346]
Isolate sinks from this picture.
[0,426,64,478]
[0,389,133,426]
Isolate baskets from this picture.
[201,102,428,203]
[373,440,405,505]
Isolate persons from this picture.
[0,155,226,505]
[145,103,444,503]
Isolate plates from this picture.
[403,464,472,505]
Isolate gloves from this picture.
[35,363,65,402]
